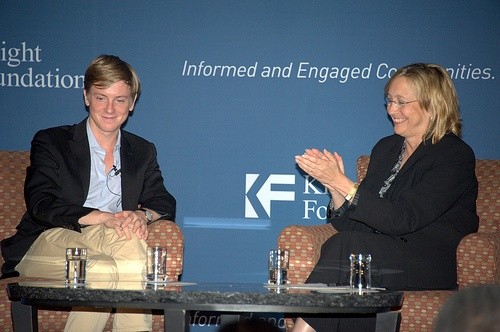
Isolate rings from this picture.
[311,168,314,172]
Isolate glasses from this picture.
[385,98,418,107]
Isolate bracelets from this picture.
[345,184,358,201]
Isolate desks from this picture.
[5,281,404,332]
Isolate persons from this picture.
[433,285,500,332]
[0,56,177,332]
[285,63,479,332]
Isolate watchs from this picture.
[137,208,153,224]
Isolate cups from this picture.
[66,247,87,284]
[268,248,290,283]
[349,251,372,291]
[147,243,167,284]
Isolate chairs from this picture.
[0,150,186,332]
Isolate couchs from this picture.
[278,155,500,332]
